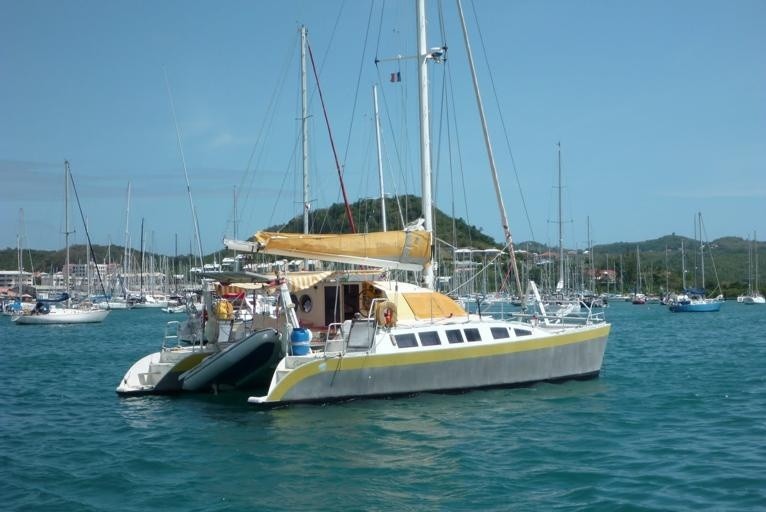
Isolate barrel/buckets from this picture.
[290,328,310,356]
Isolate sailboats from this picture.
[612,211,764,314]
[3,161,208,324]
